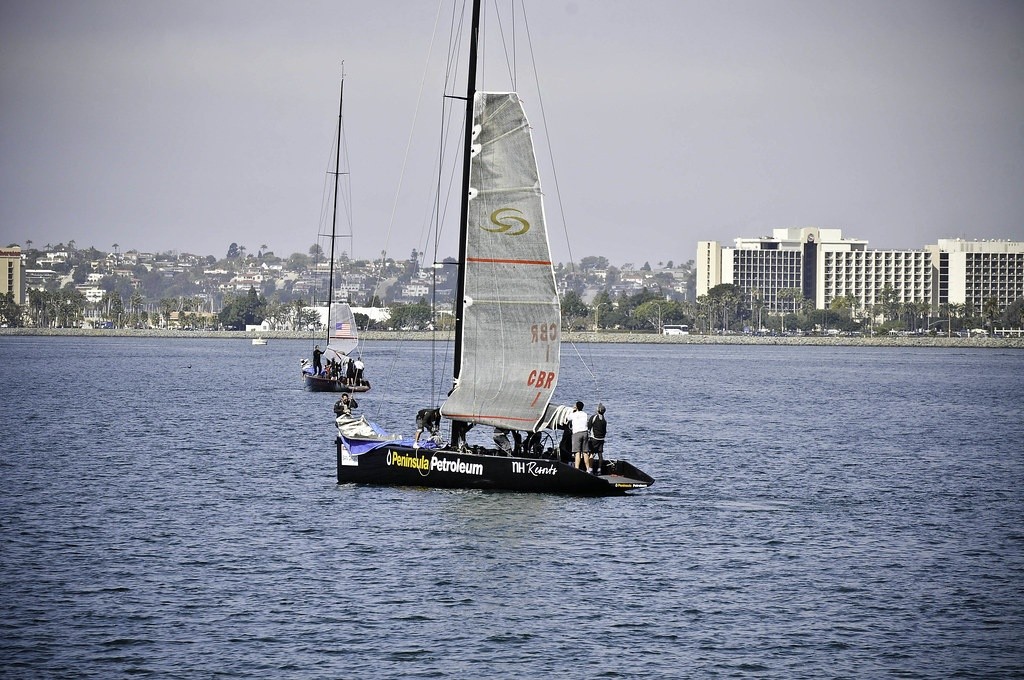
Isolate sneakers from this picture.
[413,443,419,448]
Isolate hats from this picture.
[597,403,606,414]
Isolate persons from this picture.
[334,393,358,428]
[313,345,325,376]
[588,403,607,476]
[413,408,441,449]
[325,357,365,387]
[567,402,593,475]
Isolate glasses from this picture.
[343,399,348,401]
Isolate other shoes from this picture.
[318,373,322,376]
[597,468,601,472]
[586,467,592,473]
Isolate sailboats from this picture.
[334,0,655,495]
[305,59,373,393]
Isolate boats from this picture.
[252,337,268,345]
